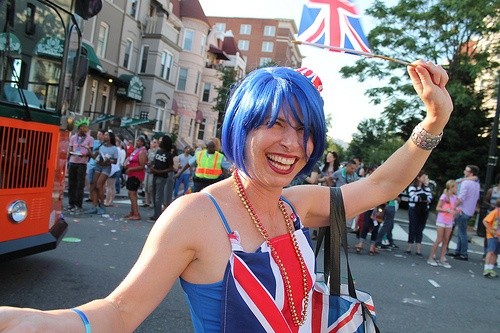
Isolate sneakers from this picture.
[438,259,452,268]
[428,258,438,267]
[89,206,106,214]
[483,270,498,277]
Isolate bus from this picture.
[0,0,102,267]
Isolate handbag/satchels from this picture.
[297,187,378,333]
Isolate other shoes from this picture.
[311,234,318,240]
[496,263,500,269]
[369,249,377,255]
[86,198,92,203]
[147,216,157,223]
[124,213,141,220]
[416,252,423,258]
[446,253,458,256]
[451,253,469,260]
[354,245,362,253]
[378,242,398,249]
[482,258,485,262]
[65,206,83,212]
[404,250,412,254]
[103,202,114,207]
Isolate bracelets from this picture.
[410,124,444,151]
[70,307,92,333]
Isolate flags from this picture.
[295,0,374,58]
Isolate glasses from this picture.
[463,170,470,173]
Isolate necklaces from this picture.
[232,169,309,328]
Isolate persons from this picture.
[0,59,454,333]
[65,122,233,223]
[297,151,400,256]
[483,178,500,278]
[427,180,461,268]
[432,165,481,261]
[404,170,433,256]
